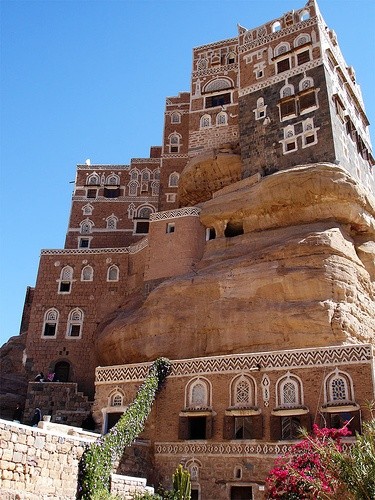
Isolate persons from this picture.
[14,403,21,420]
[32,410,40,422]
[48,371,56,382]
[35,373,43,381]
